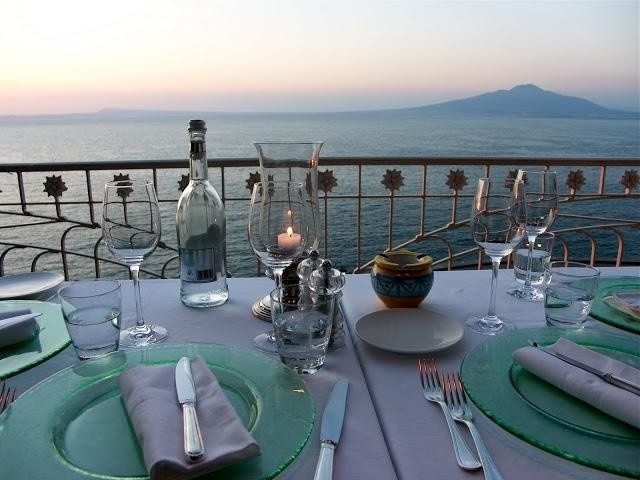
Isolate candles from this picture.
[277,232,301,251]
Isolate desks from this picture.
[1,266,397,478]
[339,267,639,476]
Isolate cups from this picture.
[270,285,335,376]
[58,279,121,360]
[543,260,600,333]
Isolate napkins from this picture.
[510,335,639,430]
[117,354,263,478]
[0,307,39,346]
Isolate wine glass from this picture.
[471,170,558,335]
[101,179,168,350]
[247,141,323,355]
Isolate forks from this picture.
[418,356,504,480]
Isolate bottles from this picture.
[297,251,346,351]
[174,119,229,309]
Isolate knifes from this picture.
[312,378,349,480]
[175,356,205,462]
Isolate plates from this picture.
[1,342,316,480]
[0,269,78,377]
[460,327,640,478]
[567,275,639,333]
[354,308,464,354]
